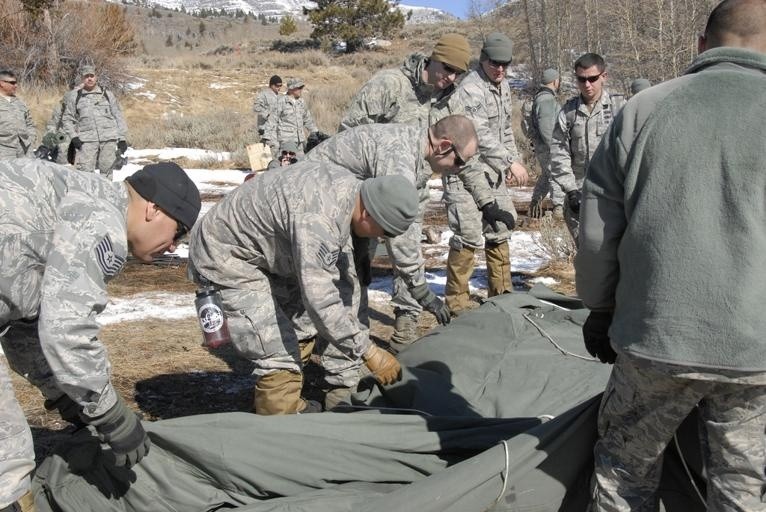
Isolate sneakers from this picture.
[551,205,564,220]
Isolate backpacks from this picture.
[521,91,553,142]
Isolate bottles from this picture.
[194,286,230,349]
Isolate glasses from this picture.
[451,144,465,166]
[282,151,295,155]
[173,221,187,241]
[442,62,461,76]
[578,72,604,82]
[4,81,16,85]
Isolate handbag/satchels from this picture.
[33,144,57,163]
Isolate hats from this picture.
[430,33,472,73]
[632,79,651,95]
[542,68,560,85]
[270,75,282,84]
[287,77,304,90]
[125,160,201,230]
[282,142,296,157]
[361,176,419,236]
[485,32,514,62]
[82,66,96,77]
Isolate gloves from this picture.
[482,199,516,232]
[567,189,582,214]
[77,390,150,469]
[583,311,617,364]
[408,278,451,326]
[43,393,86,429]
[118,141,127,155]
[71,138,82,149]
[42,133,55,147]
[361,342,404,386]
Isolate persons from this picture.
[1,31,673,512]
[575,0,766,512]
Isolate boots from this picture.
[526,200,546,218]
[388,310,420,355]
[485,241,514,297]
[298,337,332,389]
[255,370,322,414]
[444,246,480,315]
[324,386,352,410]
